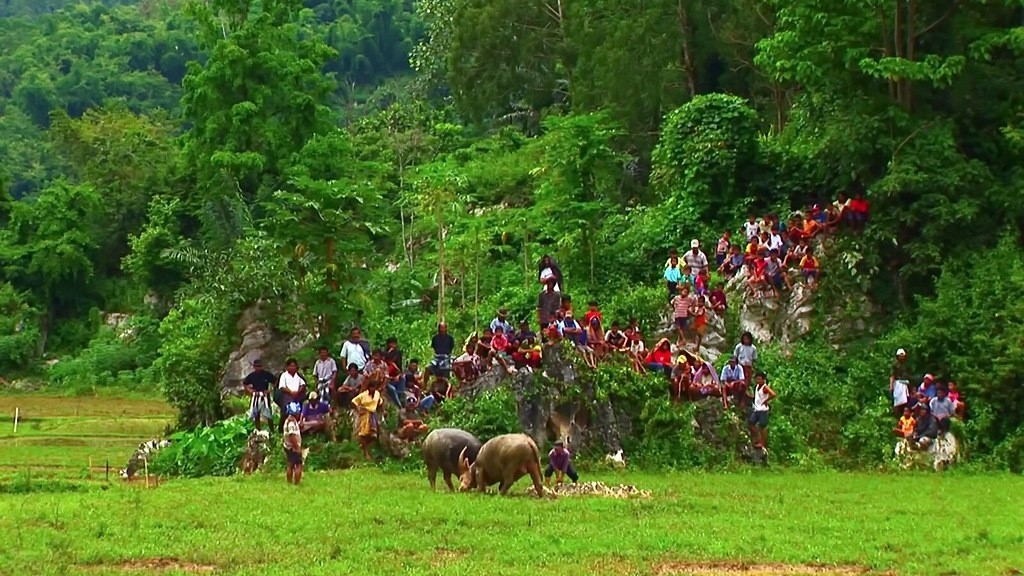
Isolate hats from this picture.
[591,318,598,324]
[677,355,687,364]
[545,274,556,282]
[497,308,508,318]
[553,440,563,448]
[309,391,318,400]
[924,374,934,381]
[896,348,905,356]
[691,239,699,248]
[729,356,736,361]
[566,310,573,318]
[253,359,263,367]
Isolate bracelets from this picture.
[270,387,274,390]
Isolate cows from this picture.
[455,431,546,499]
[420,425,483,494]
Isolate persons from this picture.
[350,381,388,461]
[880,373,966,474]
[749,372,776,448]
[538,254,565,314]
[734,331,758,391]
[720,355,747,410]
[242,359,279,433]
[889,347,914,420]
[282,402,304,485]
[278,358,307,433]
[659,185,869,309]
[313,346,340,417]
[544,440,579,488]
[337,276,723,416]
[298,392,336,442]
